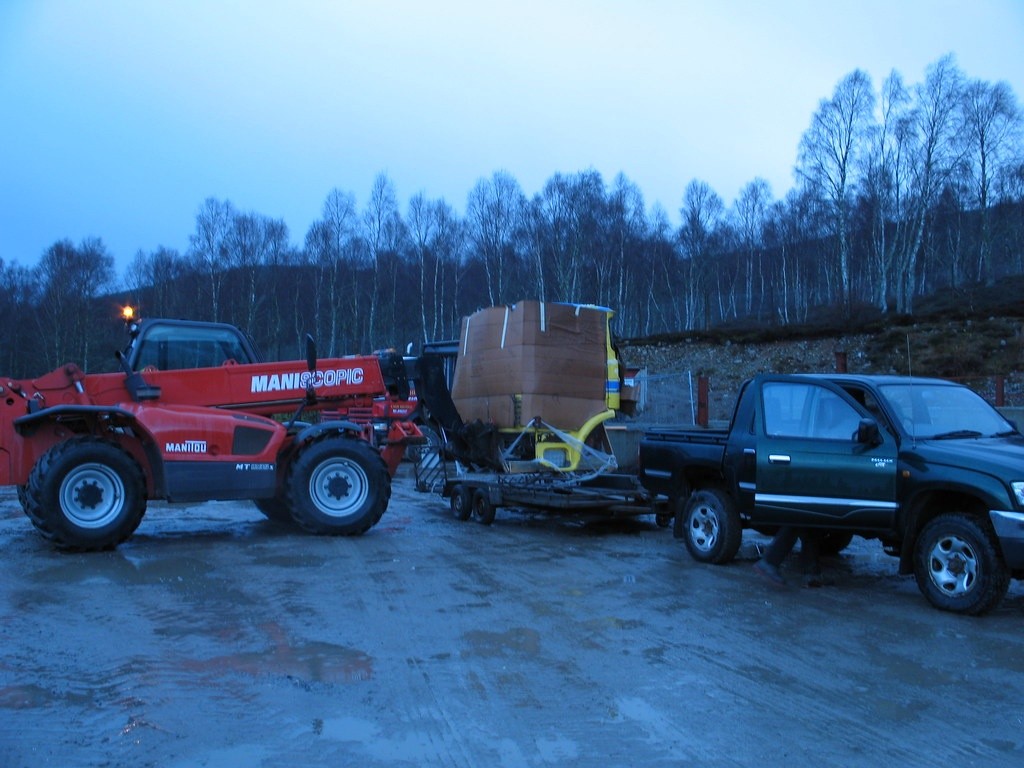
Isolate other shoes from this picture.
[755,559,789,589]
[801,559,823,588]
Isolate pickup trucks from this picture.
[644,372,1024,617]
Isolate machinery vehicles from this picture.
[0,300,496,535]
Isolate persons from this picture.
[752,408,886,589]
